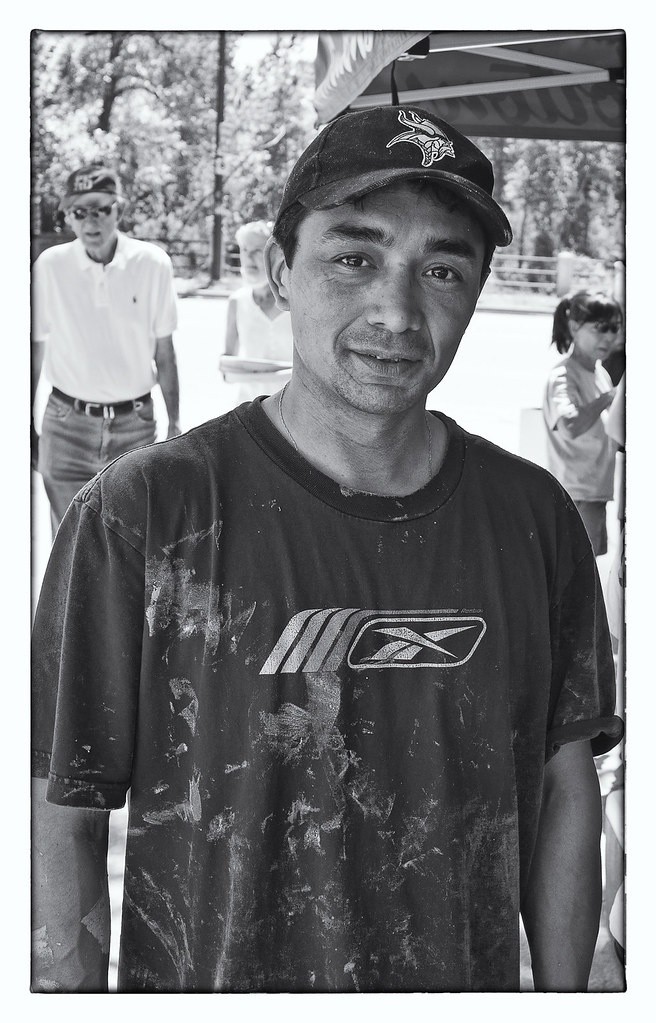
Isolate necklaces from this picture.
[279,380,435,483]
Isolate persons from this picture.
[31,164,184,549]
[29,106,627,993]
[542,291,622,557]
[216,221,293,405]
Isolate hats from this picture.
[58,166,116,210]
[273,105,512,248]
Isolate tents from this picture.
[307,30,627,142]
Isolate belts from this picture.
[52,386,151,420]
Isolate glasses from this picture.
[72,200,117,220]
[595,321,620,334]
